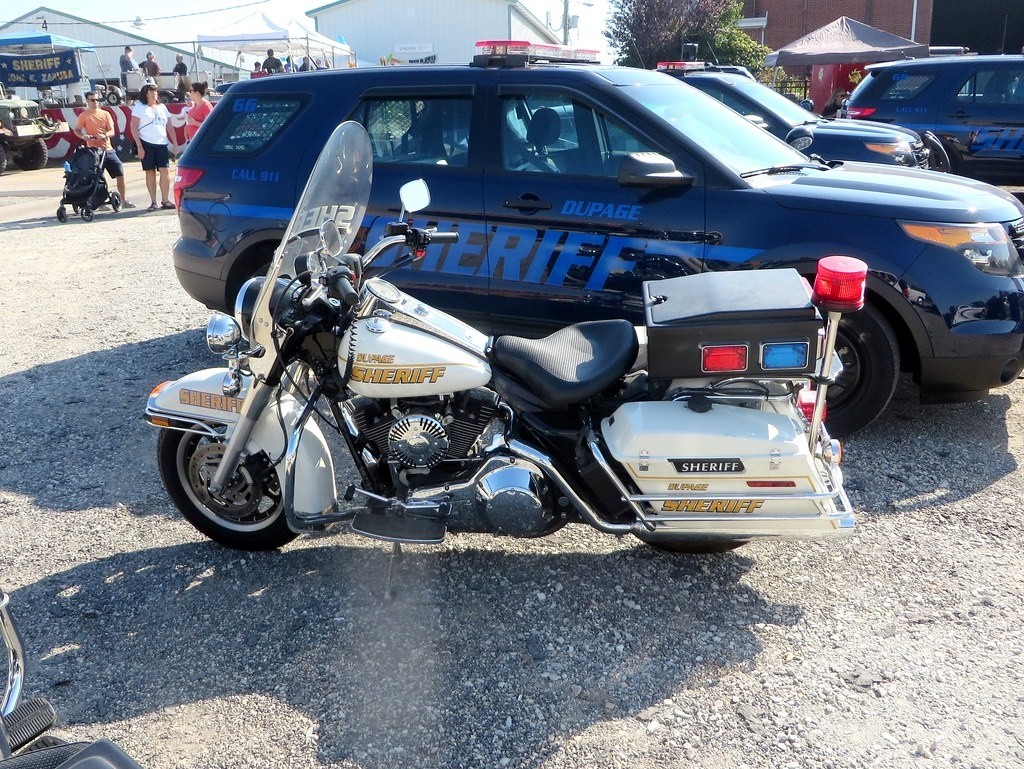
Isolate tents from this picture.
[0,29,95,55]
[197,8,350,57]
[764,15,931,66]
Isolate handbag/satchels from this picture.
[131,138,144,156]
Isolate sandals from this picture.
[147,203,158,211]
[160,201,175,209]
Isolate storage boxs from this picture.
[603,404,843,537]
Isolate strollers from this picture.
[57,137,122,224]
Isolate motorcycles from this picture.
[143,121,869,552]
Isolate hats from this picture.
[137,76,158,91]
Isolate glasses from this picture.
[88,99,99,103]
[189,89,198,93]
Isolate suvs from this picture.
[836,54,1024,193]
[0,82,61,175]
[172,39,1024,439]
[394,60,951,172]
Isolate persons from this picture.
[326,59,331,69]
[139,50,162,90]
[173,54,191,91]
[261,49,286,73]
[119,47,138,87]
[74,90,138,212]
[131,84,178,209]
[284,56,299,73]
[177,81,215,146]
[251,62,265,78]
[298,58,312,71]
[316,60,320,67]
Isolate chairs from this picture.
[984,71,1010,103]
[514,109,561,173]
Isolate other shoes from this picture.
[122,200,136,208]
[96,205,112,210]
